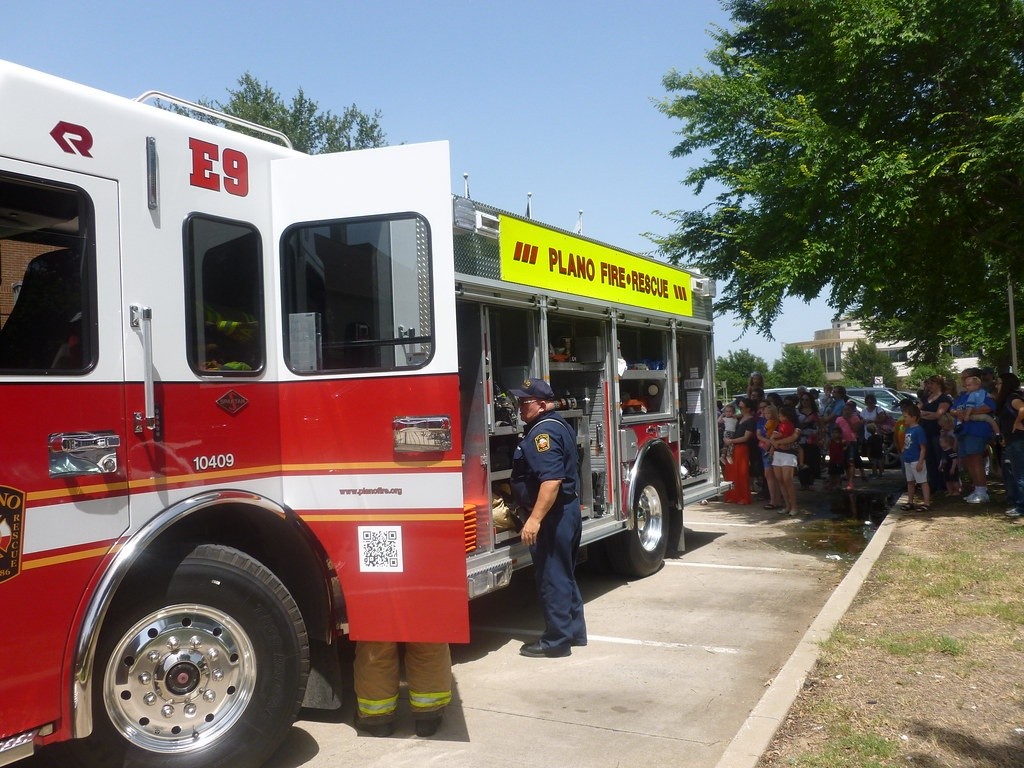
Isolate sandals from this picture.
[916,506,930,512]
[900,503,914,510]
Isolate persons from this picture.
[509,377,587,658]
[699,372,887,516]
[353,641,452,738]
[893,367,1024,517]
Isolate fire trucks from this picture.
[0,58,737,768]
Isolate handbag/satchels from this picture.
[876,407,895,434]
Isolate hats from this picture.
[508,378,554,400]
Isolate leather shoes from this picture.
[519,641,572,656]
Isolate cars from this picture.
[721,387,919,469]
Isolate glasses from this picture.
[517,398,540,406]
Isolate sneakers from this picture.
[963,494,990,503]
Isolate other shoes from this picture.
[764,503,777,508]
[995,434,1003,447]
[954,424,963,434]
[415,716,441,737]
[789,510,798,515]
[766,456,772,464]
[778,509,787,514]
[726,456,733,464]
[799,472,882,491]
[1004,508,1024,516]
[719,457,726,465]
[354,711,393,737]
